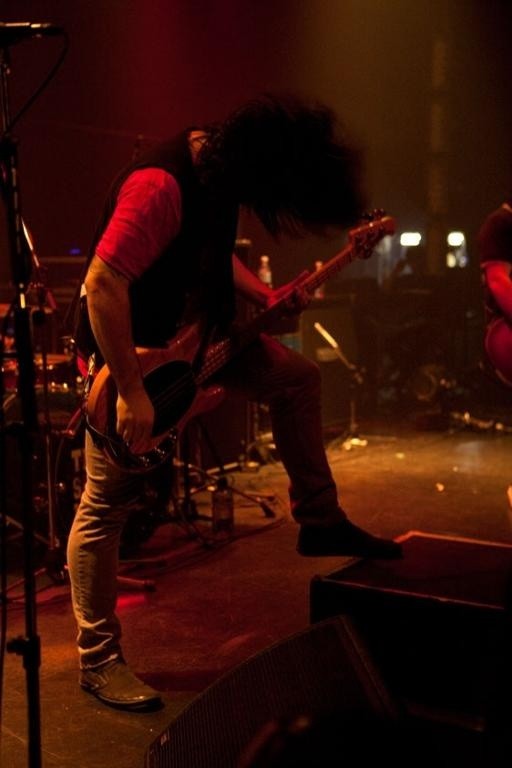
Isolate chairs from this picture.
[144,614,454,768]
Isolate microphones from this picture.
[0,21,58,48]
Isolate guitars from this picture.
[86,207,399,472]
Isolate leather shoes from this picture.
[78,656,163,712]
[295,516,406,562]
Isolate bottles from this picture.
[312,259,326,299]
[210,475,234,533]
[257,253,273,294]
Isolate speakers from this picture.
[316,384,352,427]
[308,530,512,712]
[147,613,413,767]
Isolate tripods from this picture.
[178,398,275,522]
[158,420,227,548]
[1,369,156,594]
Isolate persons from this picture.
[475,201,512,381]
[66,96,401,712]
